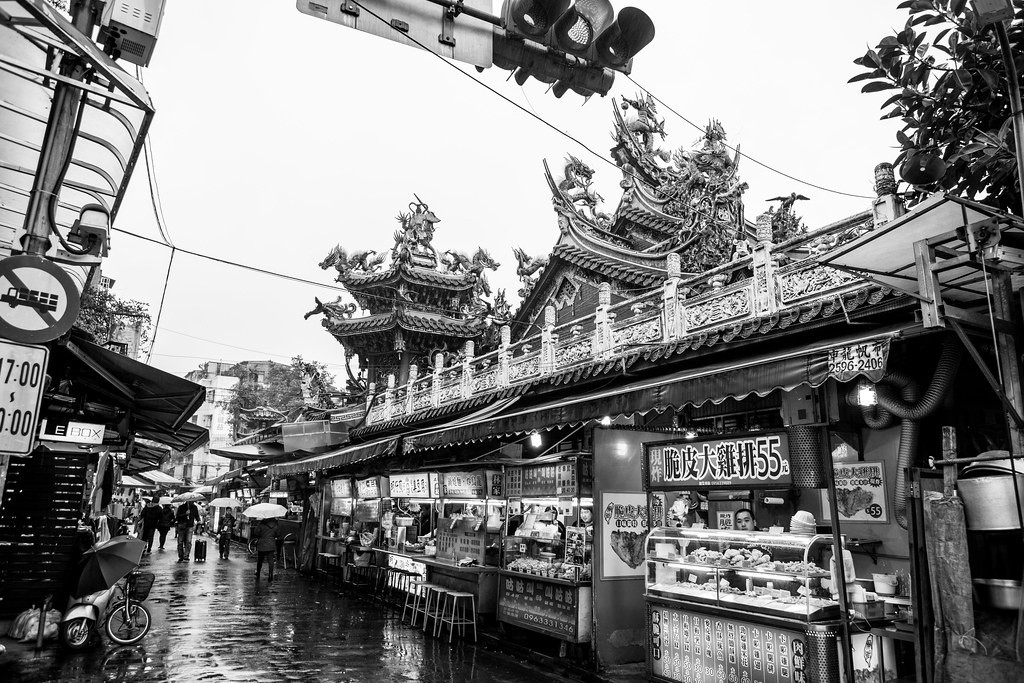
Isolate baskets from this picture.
[130,571,155,602]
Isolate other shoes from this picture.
[225,557,228,560]
[179,555,190,561]
[220,555,223,558]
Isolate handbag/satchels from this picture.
[216,531,222,540]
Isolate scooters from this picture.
[55,582,118,651]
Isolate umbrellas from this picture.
[64,535,147,601]
[241,502,288,519]
[170,492,206,519]
[207,498,243,523]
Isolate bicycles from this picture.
[103,568,155,645]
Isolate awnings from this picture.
[402,315,930,452]
[201,452,269,490]
[69,325,210,477]
[266,393,521,485]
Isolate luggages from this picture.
[195,524,207,562]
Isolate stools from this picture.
[376,566,422,602]
[346,563,378,587]
[316,553,342,586]
[402,581,476,643]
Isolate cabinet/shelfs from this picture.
[642,527,896,683]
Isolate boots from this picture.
[268,575,273,582]
[255,575,260,581]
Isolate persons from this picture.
[722,508,772,594]
[216,507,239,560]
[109,486,210,563]
[248,517,284,583]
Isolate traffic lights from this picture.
[504,0,656,75]
